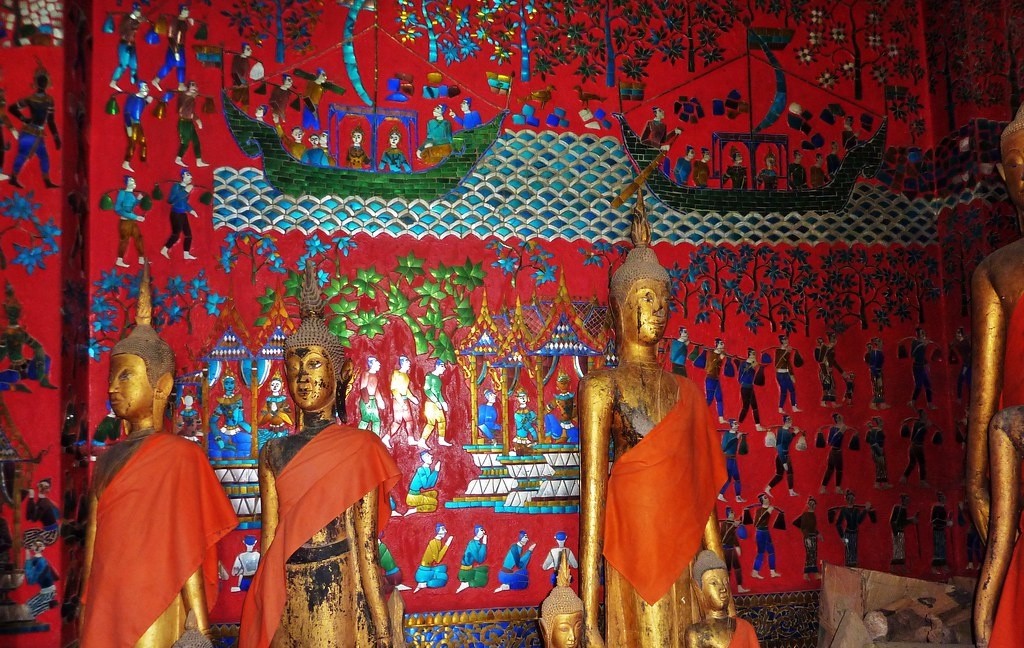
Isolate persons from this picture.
[539,184,762,648]
[71,261,238,648]
[966,100,1024,648]
[243,262,395,648]
